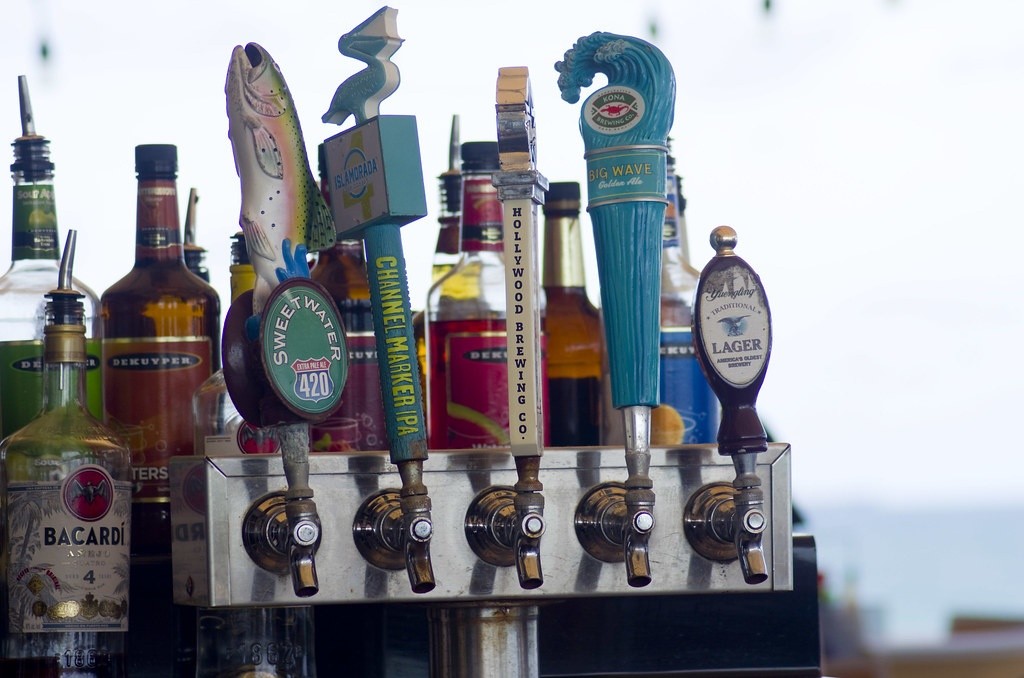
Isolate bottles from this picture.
[541,181,603,447]
[0,229,133,678]
[422,142,552,451]
[191,232,350,678]
[649,138,724,444]
[0,74,104,439]
[180,185,209,285]
[98,145,223,584]
[308,238,387,455]
[415,113,467,430]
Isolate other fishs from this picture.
[222,41,338,317]
